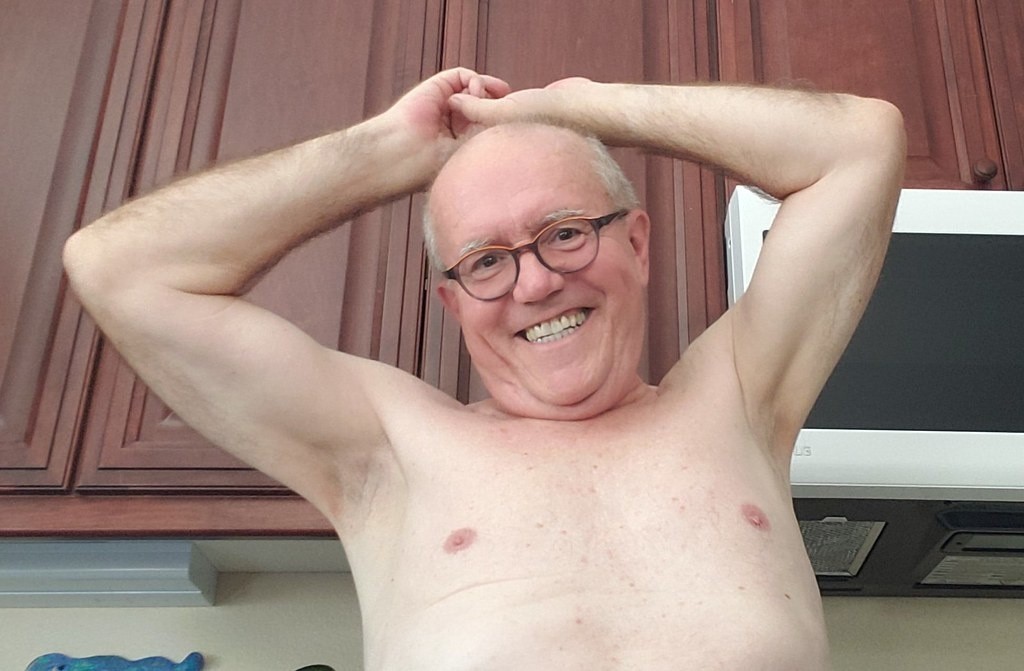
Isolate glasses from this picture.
[443,209,628,300]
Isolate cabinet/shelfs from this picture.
[0,0,1022,542]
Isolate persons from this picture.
[61,67,909,670]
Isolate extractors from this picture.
[791,497,1024,598]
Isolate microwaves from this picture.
[722,183,1024,502]
